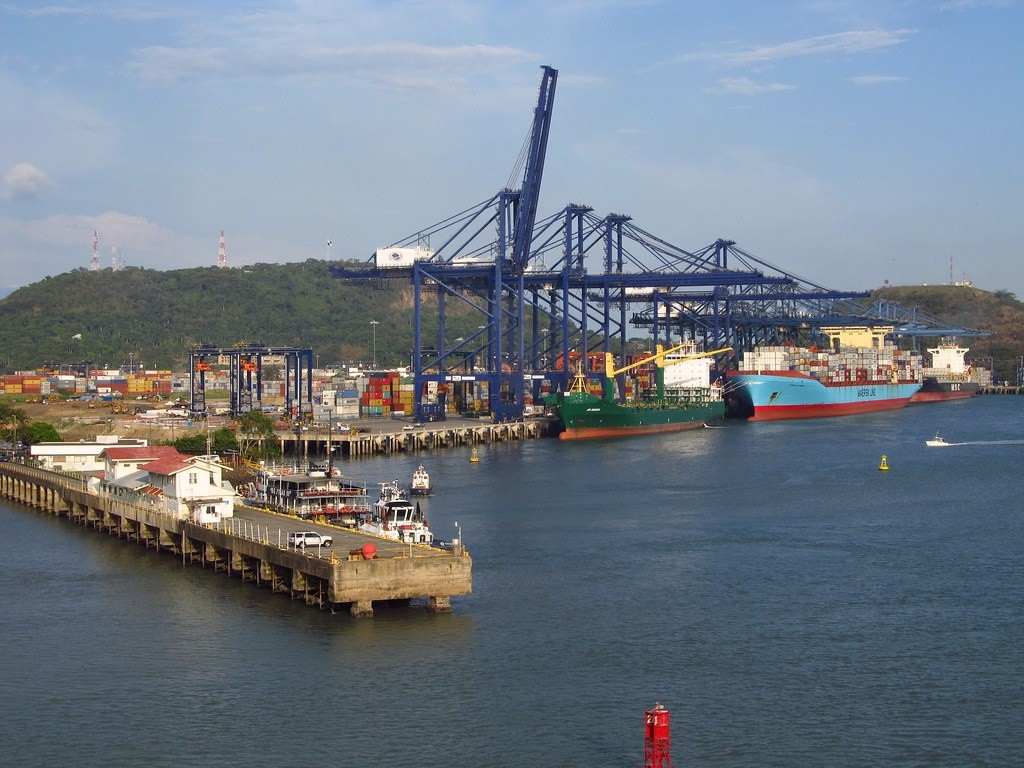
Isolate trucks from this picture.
[523,406,544,417]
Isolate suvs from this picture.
[290,530,333,548]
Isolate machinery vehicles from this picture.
[25,395,147,416]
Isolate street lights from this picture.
[316,355,319,369]
[574,338,580,370]
[369,320,379,371]
[479,325,486,372]
[129,352,134,374]
[155,382,158,395]
[541,329,549,370]
[315,397,321,420]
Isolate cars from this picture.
[332,416,341,422]
[547,405,561,418]
[414,421,425,427]
[340,424,351,431]
[356,426,369,433]
[313,422,327,428]
[301,426,309,431]
[291,427,303,434]
[326,423,335,431]
[425,412,446,422]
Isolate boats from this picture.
[409,462,434,497]
[540,343,733,440]
[357,480,433,545]
[907,335,980,403]
[925,430,951,447]
[722,344,924,422]
[234,459,373,529]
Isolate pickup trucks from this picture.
[402,424,414,430]
